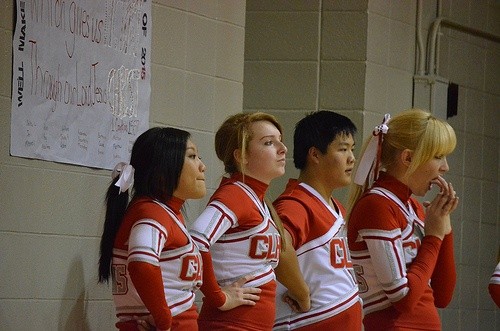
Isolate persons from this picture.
[95,126,205,330]
[347,110,458,330]
[187,112,288,331]
[272,110,365,330]
